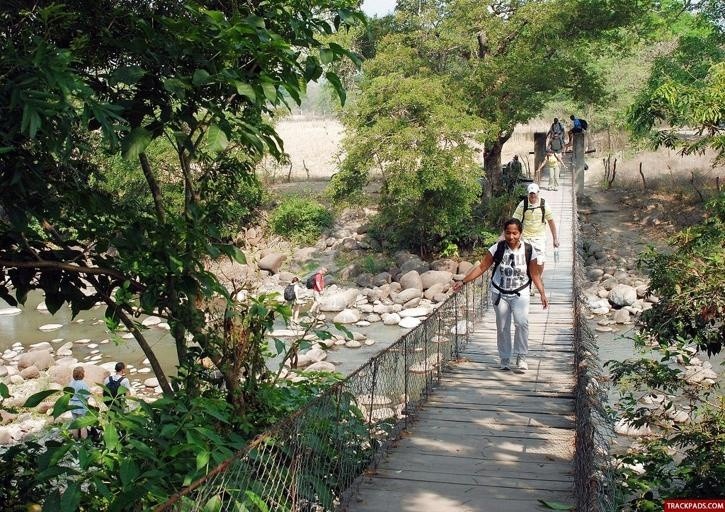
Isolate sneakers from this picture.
[500,358,511,370]
[515,355,528,370]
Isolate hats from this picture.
[526,183,539,194]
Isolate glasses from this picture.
[509,253,516,269]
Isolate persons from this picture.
[68,366,93,421]
[449,218,550,372]
[101,362,133,417]
[305,266,328,319]
[545,115,593,154]
[506,154,524,180]
[536,148,568,192]
[510,183,561,296]
[288,276,304,328]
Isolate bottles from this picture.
[555,247,560,265]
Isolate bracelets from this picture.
[461,279,466,285]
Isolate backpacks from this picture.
[306,273,319,288]
[284,283,296,302]
[574,119,587,130]
[103,376,125,411]
